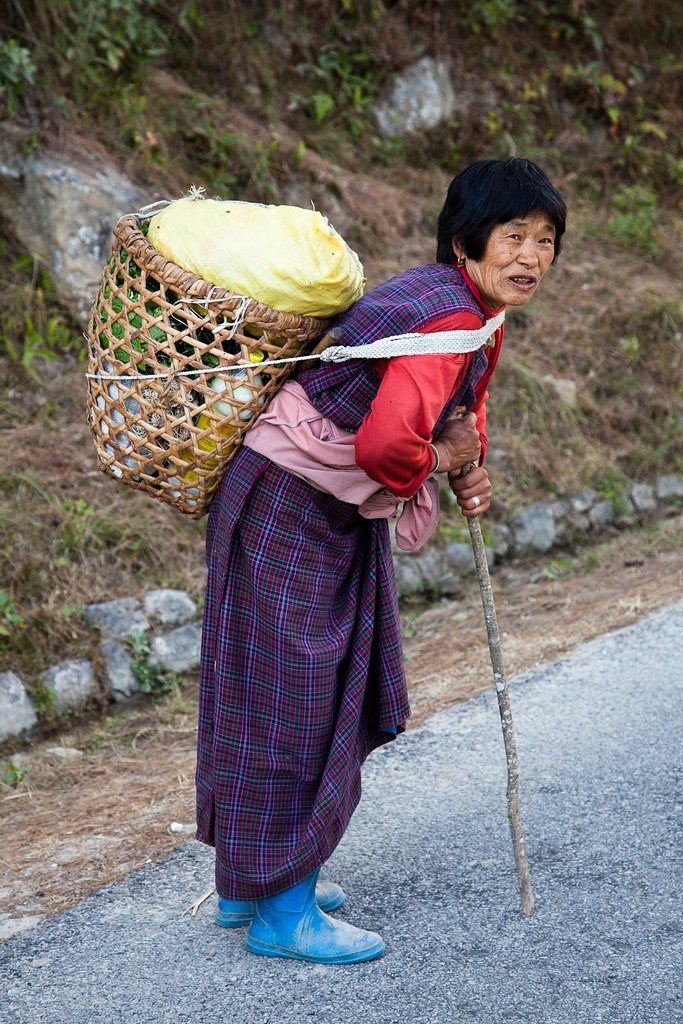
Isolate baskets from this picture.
[84,212,333,521]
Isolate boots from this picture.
[244,866,386,965]
[215,881,347,928]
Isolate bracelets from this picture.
[429,444,439,475]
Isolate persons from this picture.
[199,156,567,964]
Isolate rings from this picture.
[471,496,480,508]
